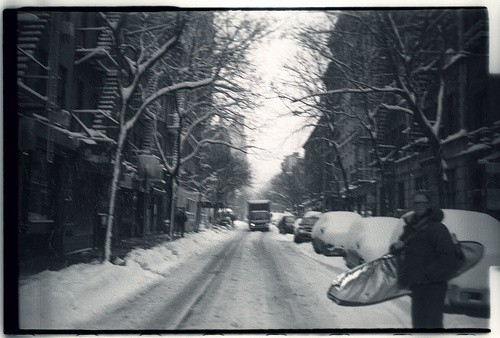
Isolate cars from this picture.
[278,209,499,315]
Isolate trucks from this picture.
[247,199,273,232]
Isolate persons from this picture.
[387,191,465,327]
[174,208,188,239]
